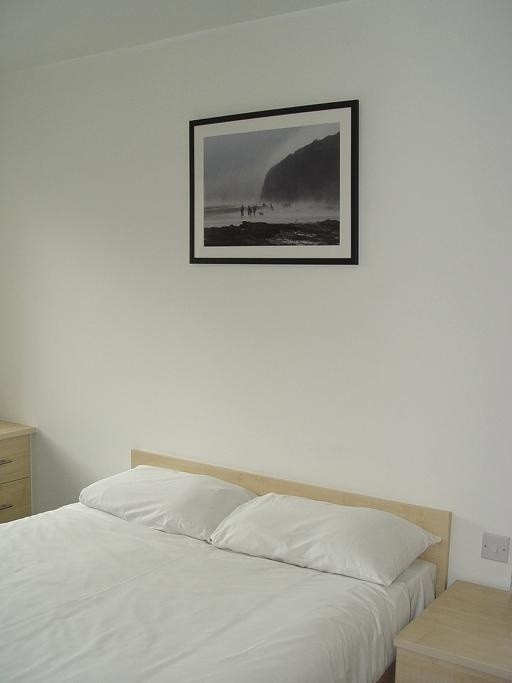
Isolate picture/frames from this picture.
[189,101,360,265]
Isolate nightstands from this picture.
[0,420,35,523]
[393,580,512,683]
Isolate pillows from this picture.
[209,492,443,587]
[79,464,260,554]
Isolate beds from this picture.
[0,449,451,683]
